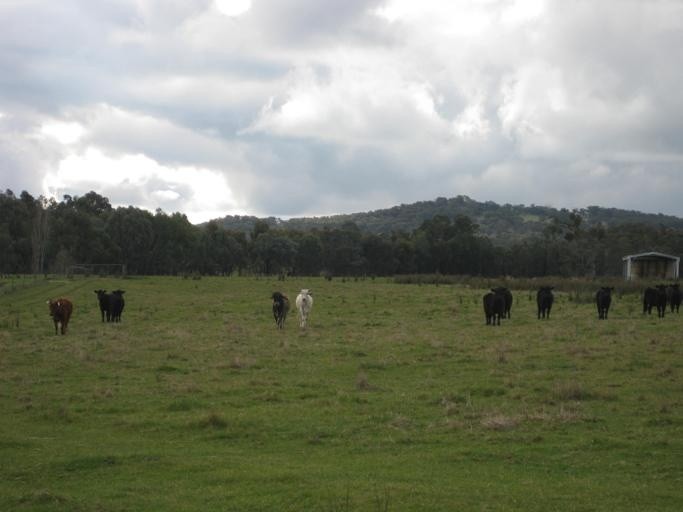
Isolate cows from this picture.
[596,287,614,320]
[94,289,126,323]
[483,286,513,326]
[666,283,683,314]
[537,286,554,320]
[269,292,290,329]
[296,289,313,328]
[46,298,73,336]
[643,284,668,318]
